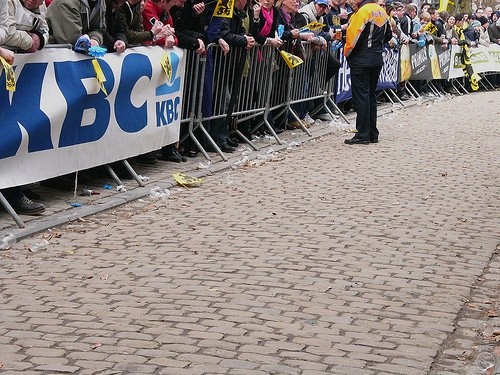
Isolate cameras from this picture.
[149,16,158,25]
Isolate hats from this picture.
[386,3,395,14]
[393,1,404,11]
[315,0,328,7]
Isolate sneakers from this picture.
[345,136,370,144]
[6,193,46,214]
[371,139,378,143]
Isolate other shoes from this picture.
[135,103,348,162]
[376,89,410,105]
[422,81,494,91]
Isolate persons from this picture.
[343,0,393,145]
[0,0,500,215]
